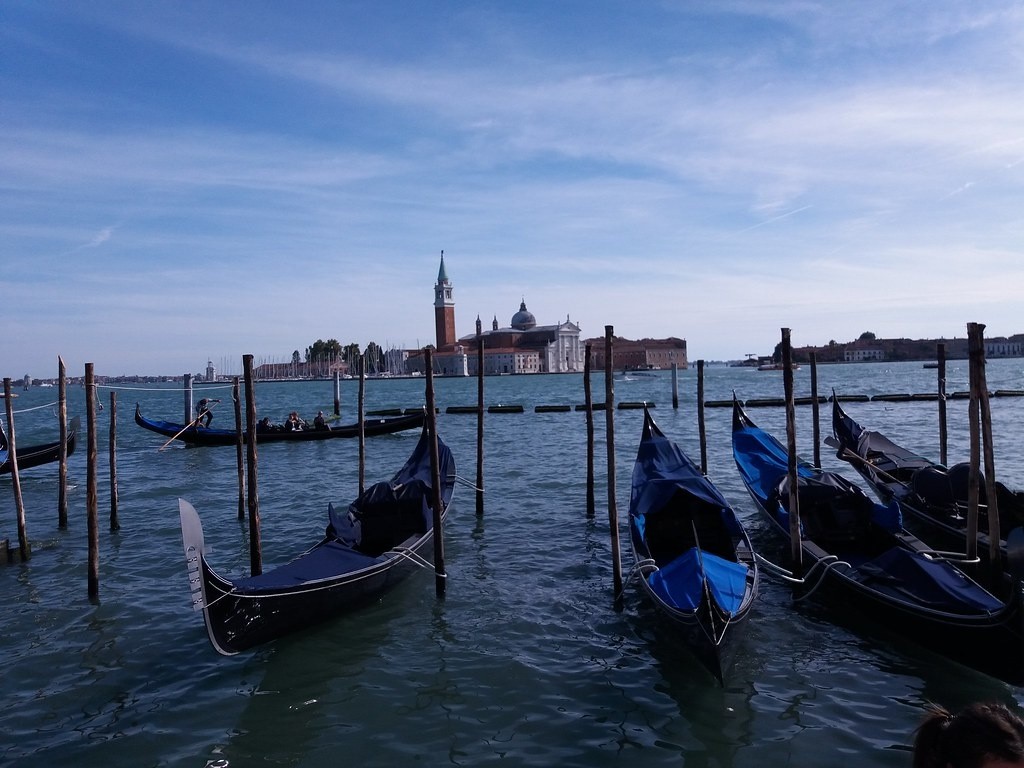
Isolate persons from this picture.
[314,411,324,431]
[196,398,221,429]
[264,412,305,431]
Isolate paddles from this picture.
[155,400,221,453]
[822,434,912,493]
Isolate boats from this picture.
[831,386,1024,601]
[0,393,19,398]
[134,401,430,445]
[730,389,1009,647]
[0,428,77,475]
[628,402,759,688]
[757,363,799,371]
[178,403,458,656]
[922,362,940,369]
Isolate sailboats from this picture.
[254,341,409,382]
